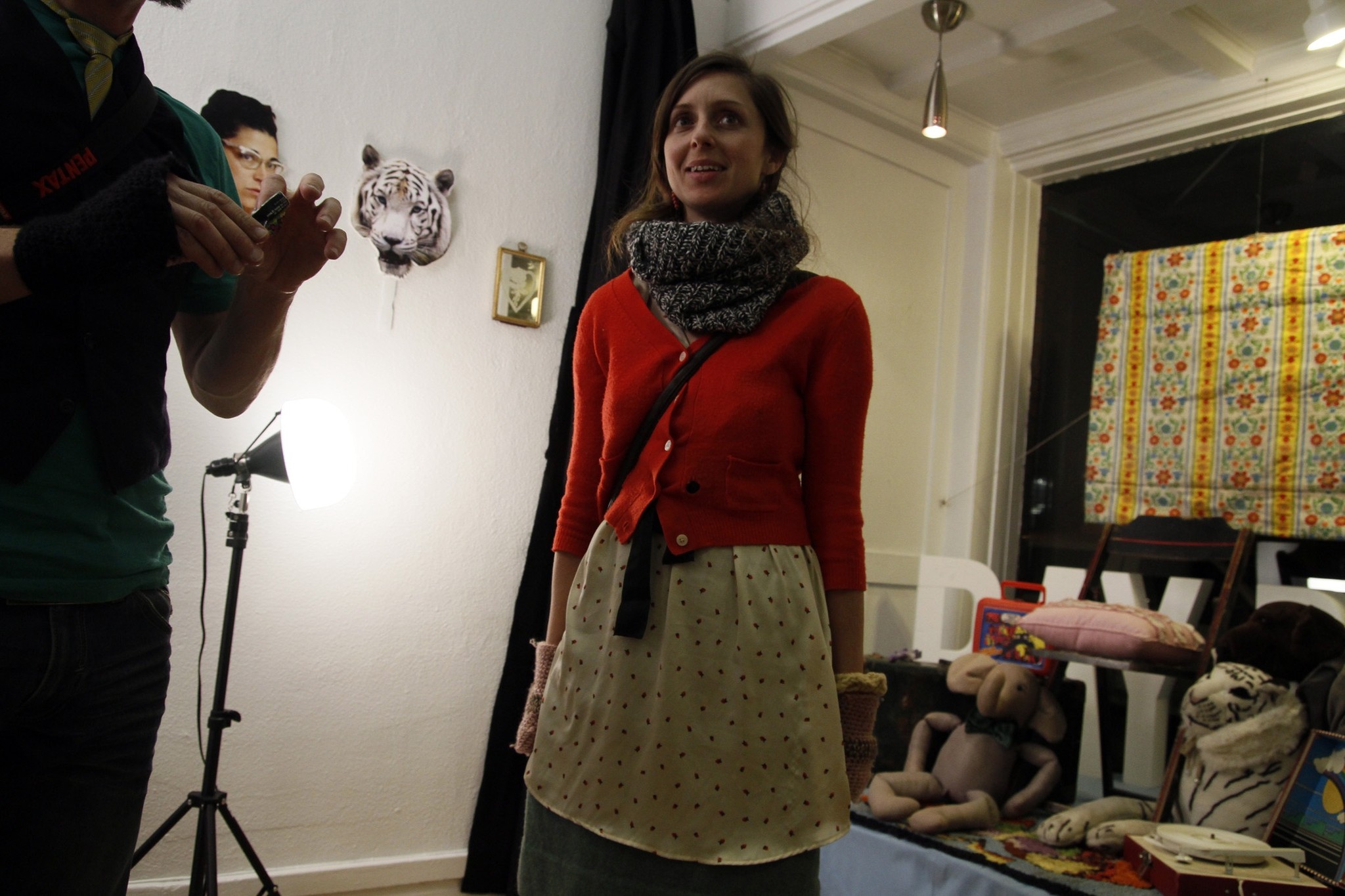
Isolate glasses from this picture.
[222,139,284,175]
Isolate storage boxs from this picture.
[1124,729,1345,895]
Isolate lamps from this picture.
[124,410,292,896]
[922,0,966,140]
[1303,0,1344,52]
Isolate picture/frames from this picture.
[492,246,546,329]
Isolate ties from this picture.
[42,0,133,123]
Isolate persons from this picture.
[514,54,874,896]
[201,87,284,217]
[0,0,346,896]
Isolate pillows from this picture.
[1016,597,1207,662]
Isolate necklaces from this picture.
[677,328,697,347]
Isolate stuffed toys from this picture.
[1039,661,1306,849]
[869,650,1059,835]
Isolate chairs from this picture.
[1026,521,1255,823]
